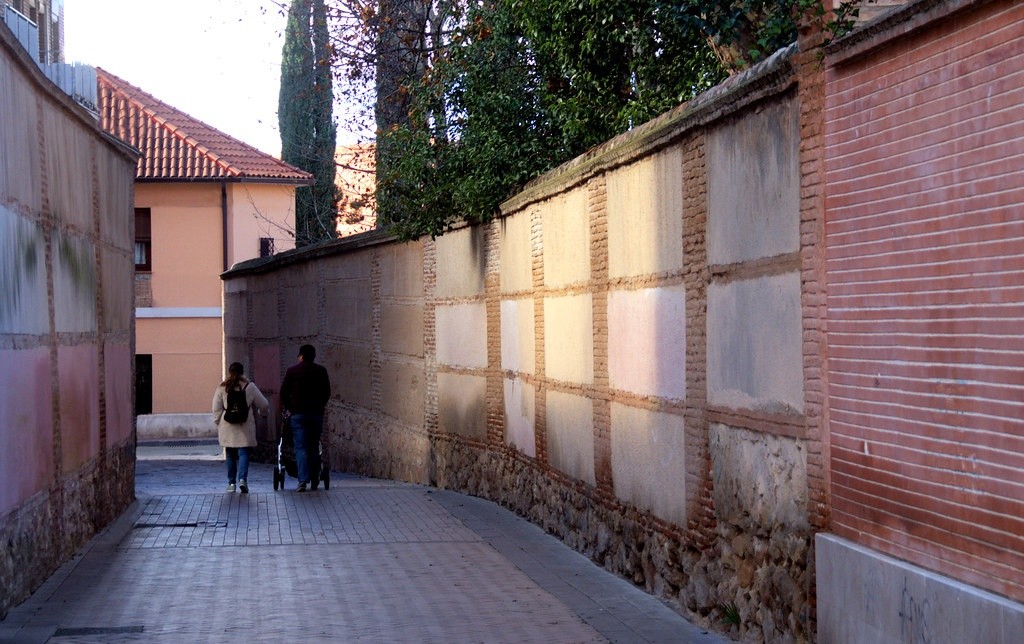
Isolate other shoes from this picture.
[298,480,319,491]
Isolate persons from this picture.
[280,344,332,492]
[212,361,269,494]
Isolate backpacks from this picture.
[220,382,251,424]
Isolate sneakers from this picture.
[226,481,250,493]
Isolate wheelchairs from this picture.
[273,414,331,492]
